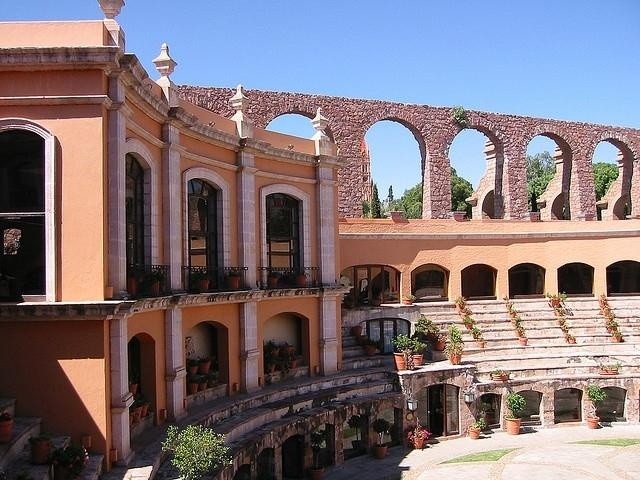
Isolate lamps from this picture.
[406,394,418,415]
[464,391,475,407]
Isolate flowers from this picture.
[51,444,91,480]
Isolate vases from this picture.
[53,464,82,480]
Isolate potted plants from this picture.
[190,266,242,293]
[540,292,577,343]
[598,360,623,375]
[268,266,312,290]
[489,367,511,382]
[373,418,391,460]
[307,430,329,480]
[353,325,382,355]
[392,313,464,370]
[587,383,609,428]
[503,392,527,435]
[127,265,167,298]
[466,417,487,439]
[403,418,433,449]
[503,295,529,347]
[0,407,58,465]
[262,338,298,372]
[402,293,417,305]
[185,356,218,394]
[347,415,362,449]
[456,297,484,349]
[597,289,623,344]
[130,381,152,425]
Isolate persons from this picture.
[340,275,353,290]
